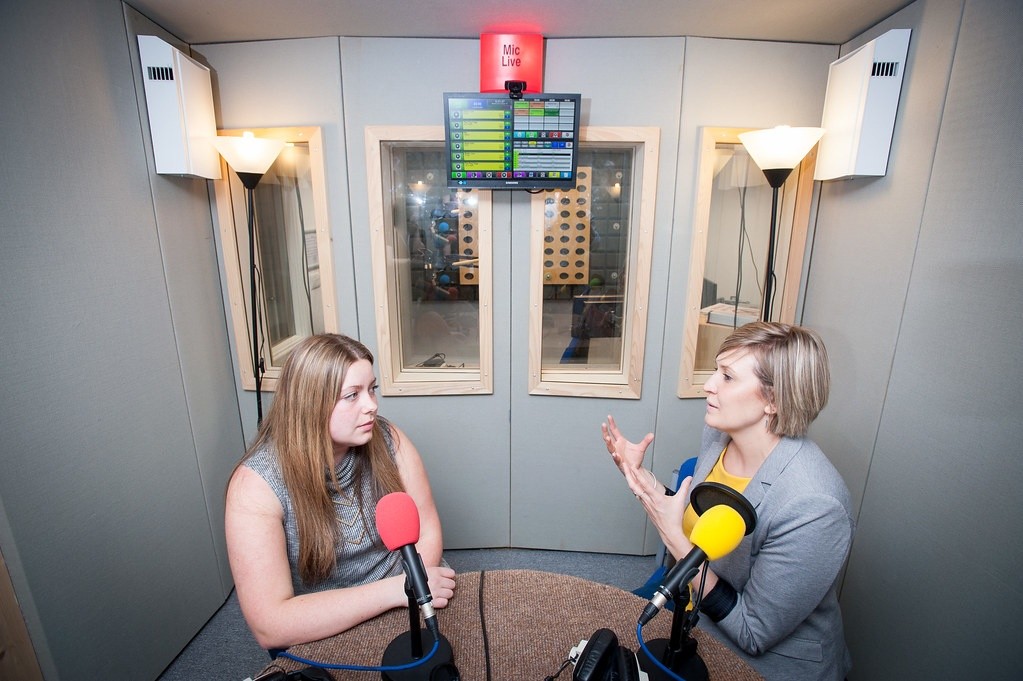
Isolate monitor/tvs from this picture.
[571,295,625,339]
[443,92,581,190]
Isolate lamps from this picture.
[740,123,830,319]
[211,132,288,426]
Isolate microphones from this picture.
[376,492,441,643]
[638,504,747,626]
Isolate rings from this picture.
[639,492,644,497]
[634,491,637,495]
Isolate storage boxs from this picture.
[707,305,760,328]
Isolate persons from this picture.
[603,322,859,681]
[224,333,455,661]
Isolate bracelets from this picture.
[646,469,656,489]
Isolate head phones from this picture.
[567,628,649,681]
